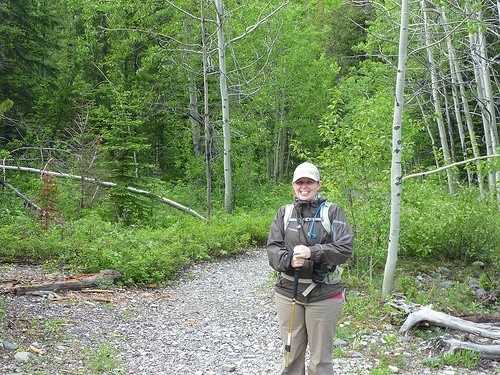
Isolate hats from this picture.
[293,162,320,182]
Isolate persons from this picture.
[267,162,353,375]
